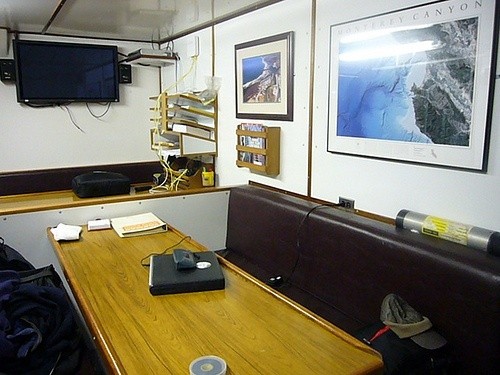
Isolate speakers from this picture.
[0,28,8,57]
[0,59,15,81]
[118,64,131,83]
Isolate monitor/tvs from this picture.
[12,39,119,103]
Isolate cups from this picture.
[189,355,227,375]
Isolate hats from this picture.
[380,294,447,350]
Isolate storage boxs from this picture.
[172,124,210,140]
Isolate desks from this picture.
[49,217,384,375]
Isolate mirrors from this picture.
[0,0,319,213]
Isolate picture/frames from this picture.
[235,32,292,117]
[327,1,499,174]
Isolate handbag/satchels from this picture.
[71,171,130,198]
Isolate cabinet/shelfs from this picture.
[149,93,215,142]
[235,125,280,174]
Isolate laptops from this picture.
[148,250,225,296]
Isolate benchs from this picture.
[216,185,500,373]
